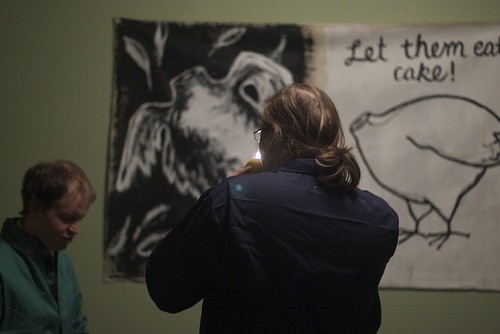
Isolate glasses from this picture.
[252,125,272,142]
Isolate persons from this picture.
[145,84,399,334]
[0,159,97,334]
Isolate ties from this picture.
[43,253,58,304]
